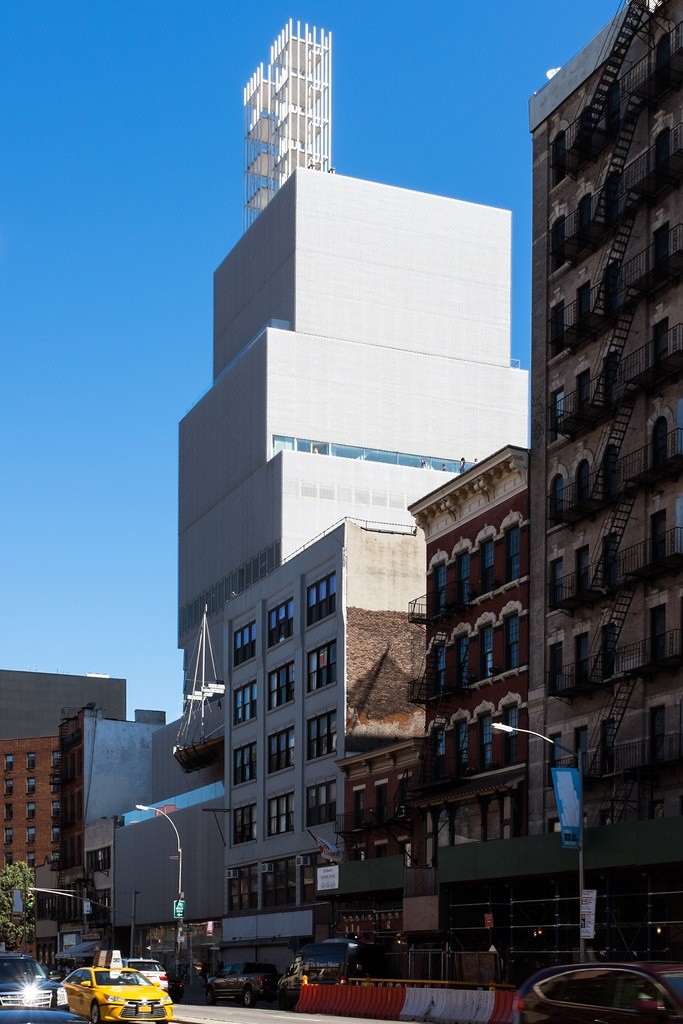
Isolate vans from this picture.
[275,938,380,1011]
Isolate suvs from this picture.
[205,960,279,1009]
[121,957,170,994]
[0,950,69,1010]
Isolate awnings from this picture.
[55,941,100,959]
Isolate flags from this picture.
[312,831,343,862]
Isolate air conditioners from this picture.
[227,870,238,879]
[296,856,310,866]
[261,863,273,873]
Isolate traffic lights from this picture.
[26,893,36,909]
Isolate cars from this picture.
[60,949,174,1024]
[0,1003,91,1024]
[511,961,683,1024]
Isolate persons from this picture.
[63,965,72,978]
[181,959,224,988]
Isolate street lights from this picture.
[490,720,588,964]
[134,804,183,980]
